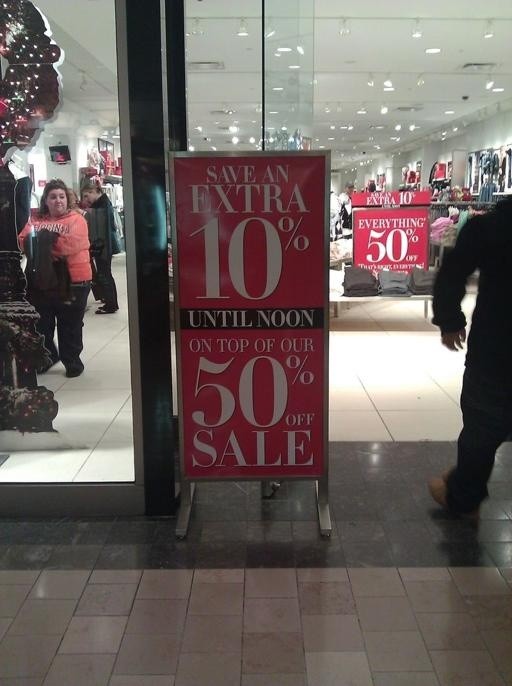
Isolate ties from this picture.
[48,144,72,164]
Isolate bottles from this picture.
[345,181,354,188]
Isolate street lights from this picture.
[90,274,102,301]
[111,228,121,254]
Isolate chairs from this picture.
[60,352,84,378]
[431,468,479,519]
[95,304,118,314]
[37,356,57,372]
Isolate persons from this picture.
[329,191,343,243]
[339,183,354,238]
[67,178,121,315]
[17,179,96,379]
[368,182,376,191]
[425,195,511,515]
